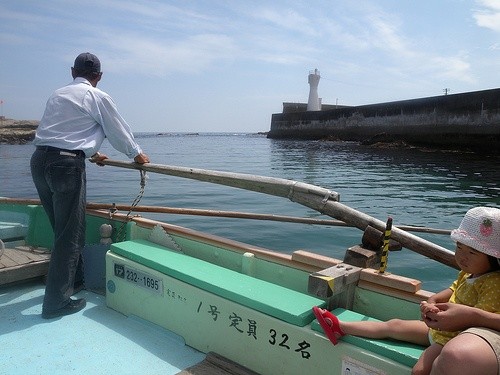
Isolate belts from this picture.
[36,146,85,158]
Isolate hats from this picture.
[450,206,500,258]
[74,52,100,73]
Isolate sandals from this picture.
[312,306,345,346]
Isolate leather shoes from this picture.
[42,298,86,319]
[74,280,83,288]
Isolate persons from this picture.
[425,303,500,375]
[312,207,500,375]
[30,52,150,319]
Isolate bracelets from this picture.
[92,152,99,159]
